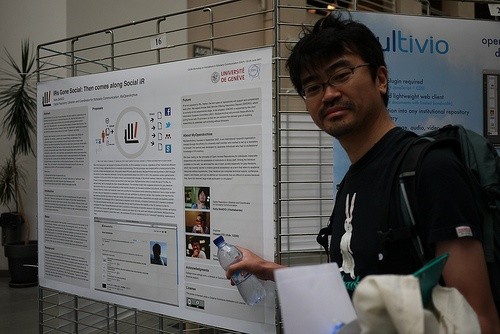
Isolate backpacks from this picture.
[395,124,500,272]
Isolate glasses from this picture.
[300,65,369,98]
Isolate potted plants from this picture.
[0,34,51,288]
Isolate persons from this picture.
[192,188,209,209]
[192,212,209,233]
[150,243,166,264]
[225,9,500,334]
[189,236,207,257]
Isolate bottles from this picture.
[213,235,267,306]
